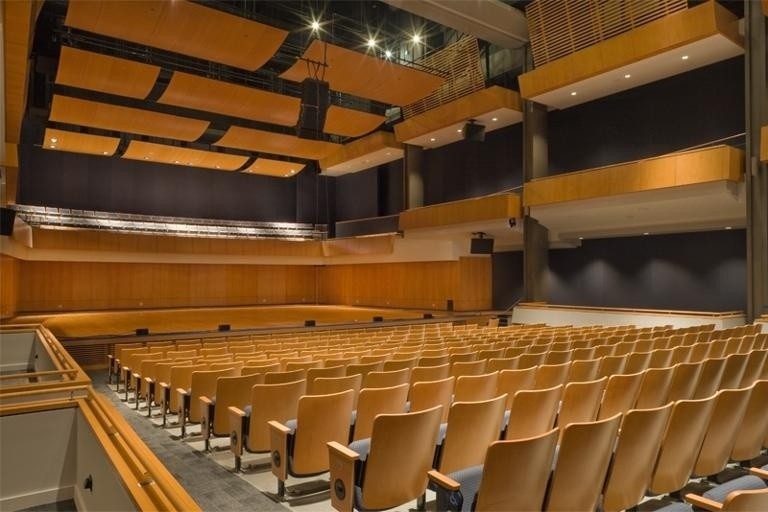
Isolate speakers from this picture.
[469,238,495,255]
[0,207,16,236]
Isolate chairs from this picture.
[108,322,768,512]
[6,201,328,240]
